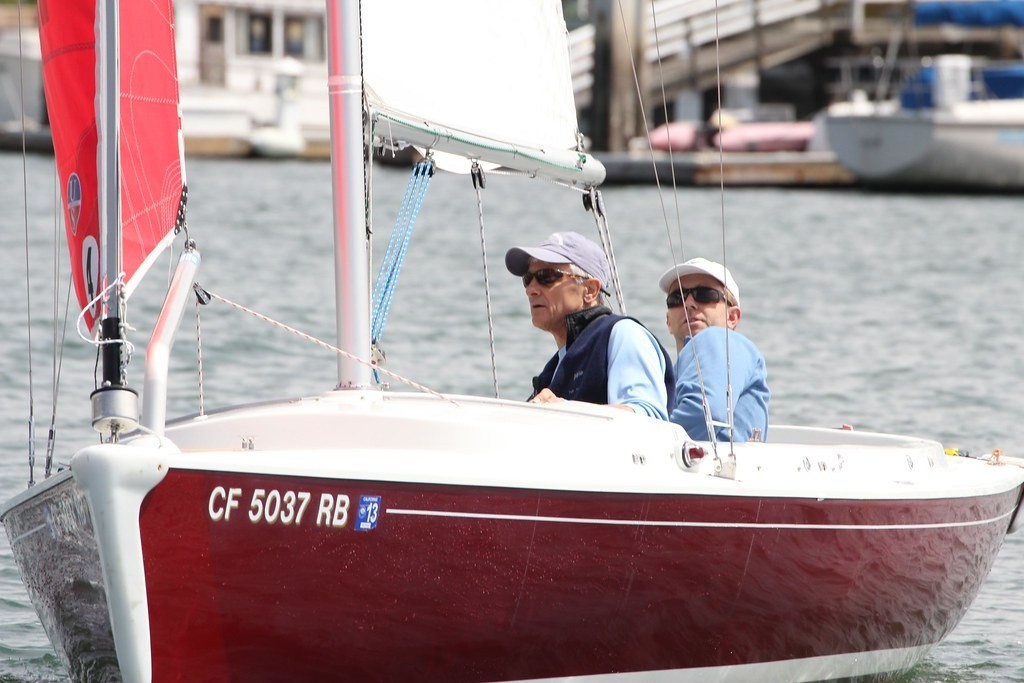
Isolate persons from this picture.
[505,231,676,420]
[659,257,771,442]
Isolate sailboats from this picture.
[0,1,1024,683]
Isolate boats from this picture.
[819,1,1024,193]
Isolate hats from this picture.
[659,258,740,307]
[505,231,610,291]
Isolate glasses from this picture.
[522,268,611,297]
[666,286,733,308]
